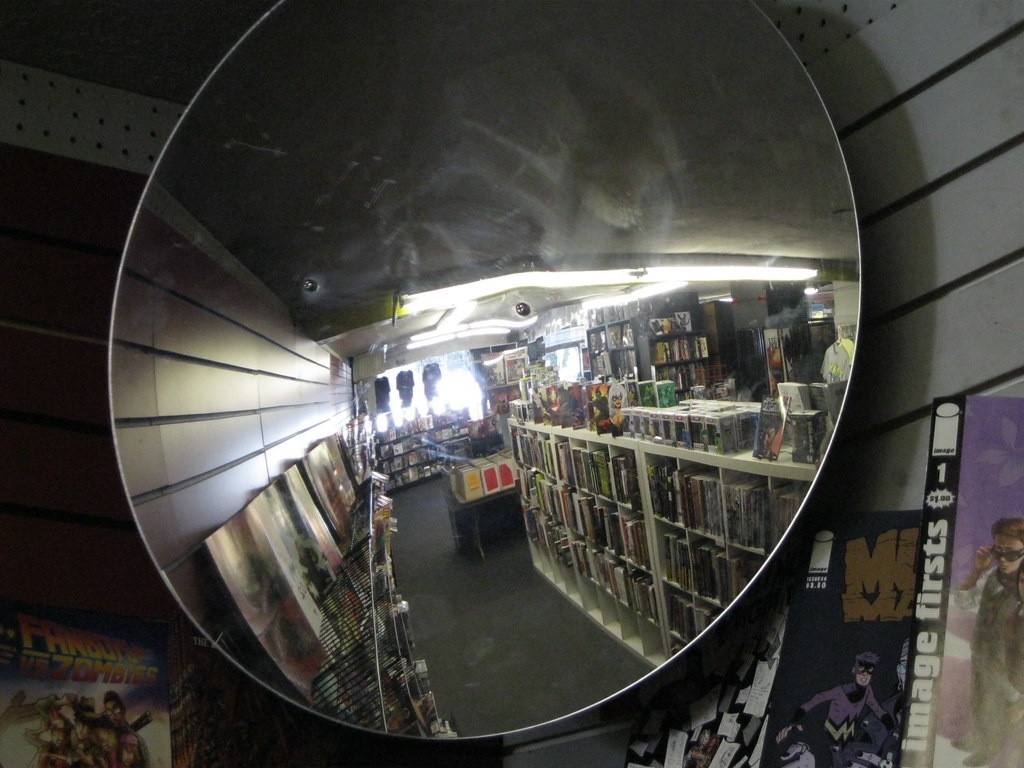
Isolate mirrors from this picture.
[107,2,864,743]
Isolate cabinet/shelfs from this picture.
[504,417,824,671]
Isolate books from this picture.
[158,277,857,739]
